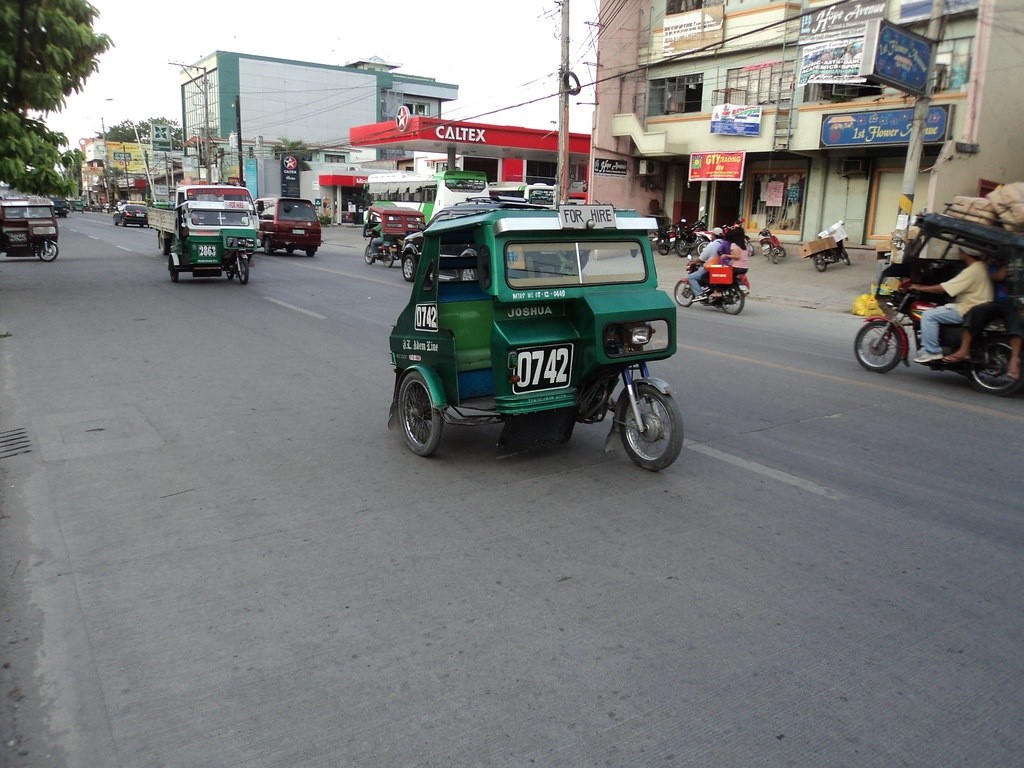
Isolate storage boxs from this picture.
[800,237,838,259]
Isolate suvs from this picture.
[400,195,589,282]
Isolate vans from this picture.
[250,197,321,257]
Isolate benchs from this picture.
[436,280,495,371]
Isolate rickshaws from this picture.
[362,206,427,268]
[386,194,685,473]
[852,202,1024,398]
[153,184,265,285]
[49,197,85,218]
[0,195,60,263]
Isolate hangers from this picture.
[763,172,799,183]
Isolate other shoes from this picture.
[691,293,708,302]
[367,250,379,257]
[709,257,732,284]
[913,352,944,364]
[710,290,722,297]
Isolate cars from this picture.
[113,203,150,228]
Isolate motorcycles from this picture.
[650,206,755,258]
[674,254,748,316]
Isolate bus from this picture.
[487,182,554,210]
[361,169,492,238]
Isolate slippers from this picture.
[942,352,971,363]
[1005,357,1021,382]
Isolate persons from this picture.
[85,202,111,214]
[688,227,749,303]
[369,216,394,255]
[418,105,425,116]
[903,243,1024,380]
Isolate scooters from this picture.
[809,222,851,272]
[757,216,786,265]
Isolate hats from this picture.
[960,245,982,257]
[708,227,723,236]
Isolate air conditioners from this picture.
[640,160,659,176]
